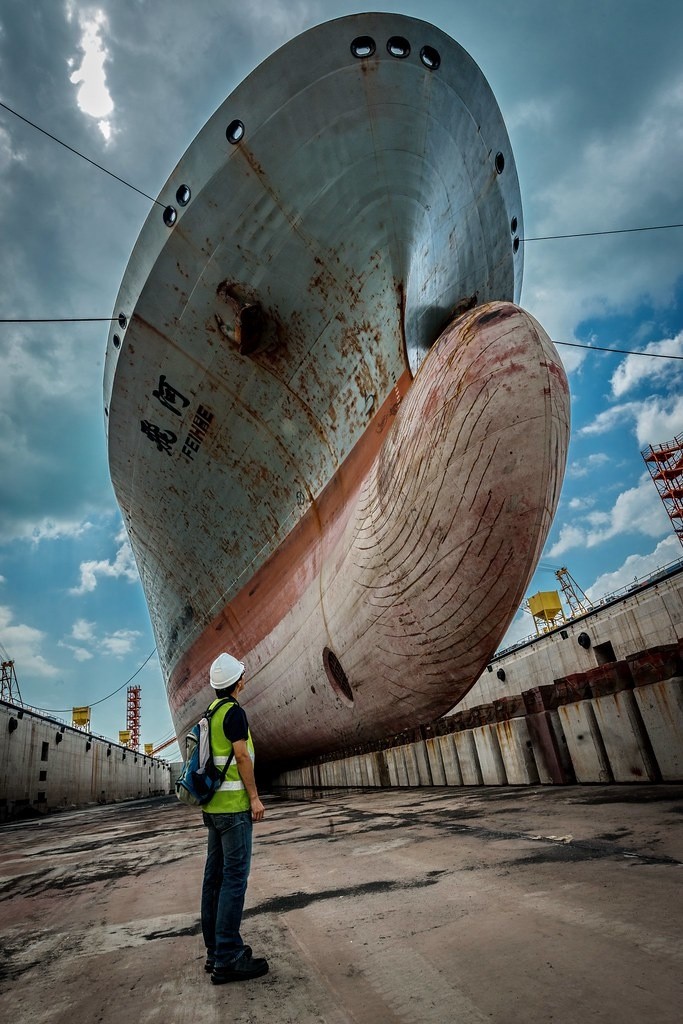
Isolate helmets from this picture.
[210,652,247,690]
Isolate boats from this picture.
[100,12,574,787]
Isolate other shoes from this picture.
[203,943,252,973]
[211,954,269,985]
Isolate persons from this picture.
[201,652,269,985]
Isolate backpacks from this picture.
[173,698,243,808]
